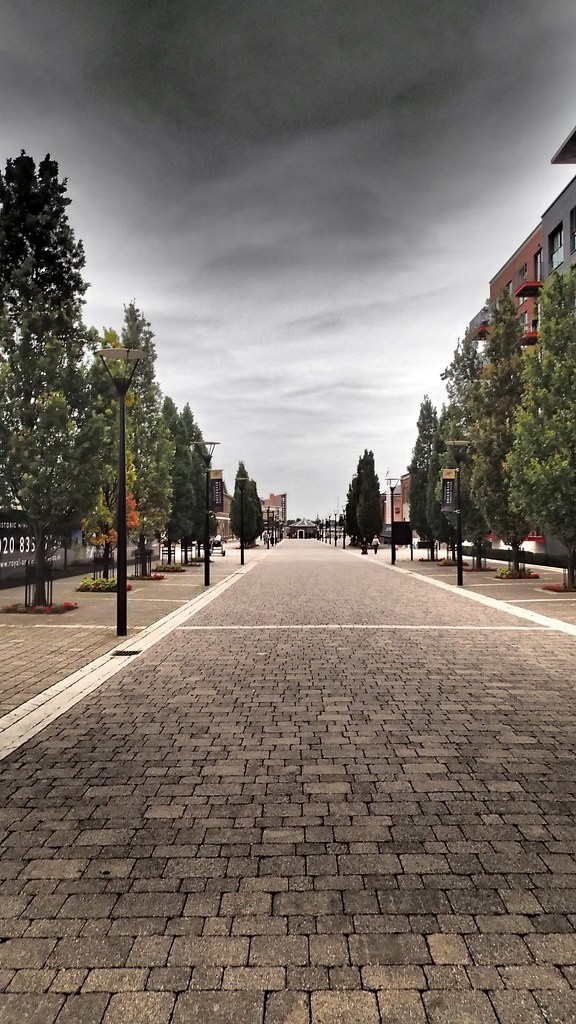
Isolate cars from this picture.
[213,535,225,547]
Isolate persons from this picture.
[361,537,368,552]
[263,532,268,544]
[371,534,380,554]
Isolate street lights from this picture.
[319,505,350,550]
[190,441,221,586]
[386,478,403,564]
[446,439,470,584]
[92,349,151,637]
[264,505,279,550]
[234,478,250,565]
